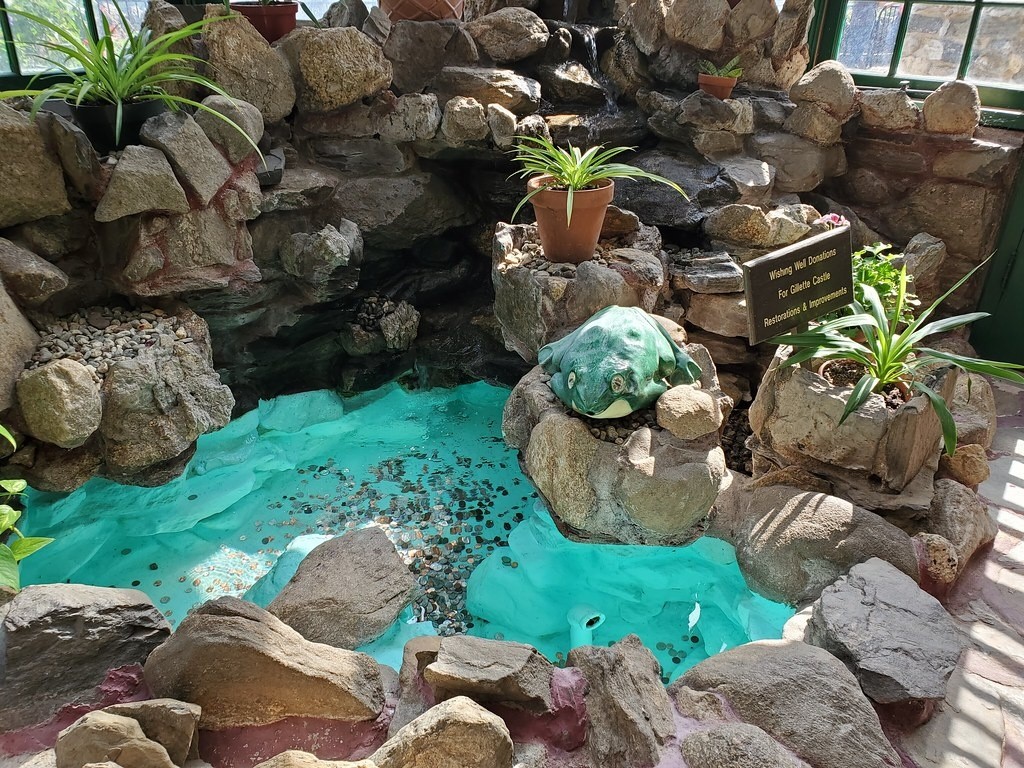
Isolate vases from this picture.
[376,0,465,23]
[229,0,298,44]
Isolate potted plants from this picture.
[762,247,1023,458]
[1,2,274,178]
[499,134,694,266]
[696,53,746,100]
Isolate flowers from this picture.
[813,211,850,234]
